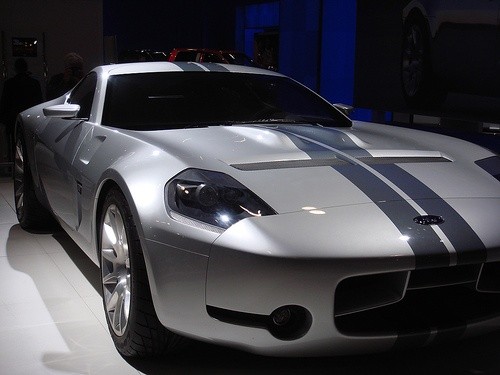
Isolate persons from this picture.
[62,53,85,83]
[3,58,43,163]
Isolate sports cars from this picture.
[11,61,500,366]
